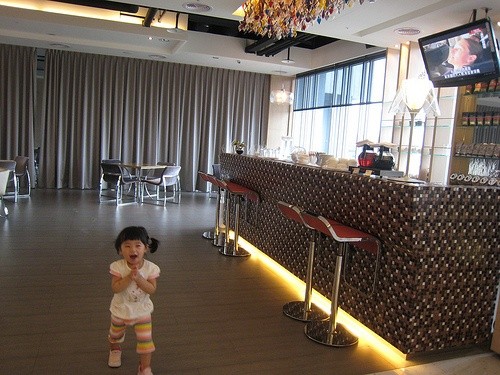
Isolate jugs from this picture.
[375,146,393,169]
[358,144,377,167]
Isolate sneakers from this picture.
[108,348,122,367]
[137,365,154,375]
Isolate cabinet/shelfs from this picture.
[447,86,500,185]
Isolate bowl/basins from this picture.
[291,152,332,165]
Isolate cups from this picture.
[468,158,497,176]
[327,158,356,169]
[260,148,279,158]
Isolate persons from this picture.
[447,37,483,75]
[108,226,161,375]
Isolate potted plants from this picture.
[232,139,247,155]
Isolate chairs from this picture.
[98,160,183,208]
[0,156,31,219]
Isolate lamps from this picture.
[270,77,294,105]
[388,79,440,183]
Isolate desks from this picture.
[120,164,168,200]
[0,159,16,174]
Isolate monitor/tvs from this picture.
[418,18,500,88]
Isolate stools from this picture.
[199,171,259,257]
[277,202,380,347]
